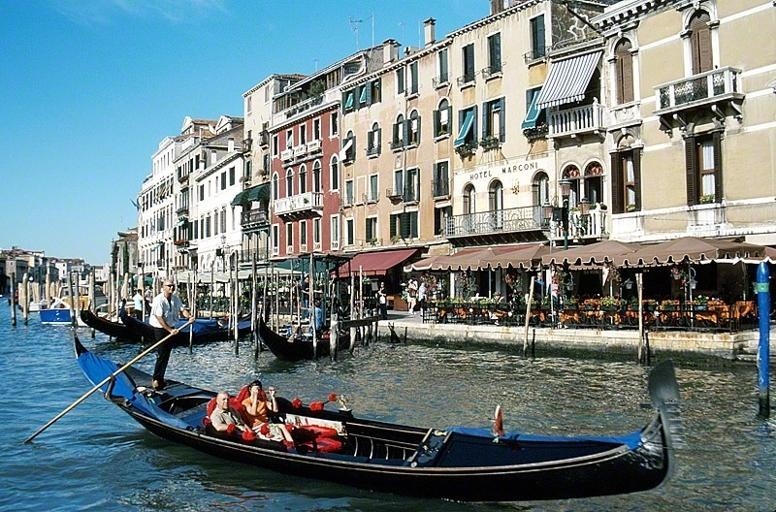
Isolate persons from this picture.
[132,289,143,321]
[241,380,295,443]
[148,279,196,389]
[308,297,323,341]
[302,274,309,319]
[418,281,426,317]
[408,279,418,316]
[145,285,153,316]
[209,390,284,443]
[377,281,387,320]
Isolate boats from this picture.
[56,327,686,504]
[15,284,130,324]
[75,308,245,343]
[116,300,272,348]
[249,306,372,357]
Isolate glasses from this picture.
[164,285,175,288]
